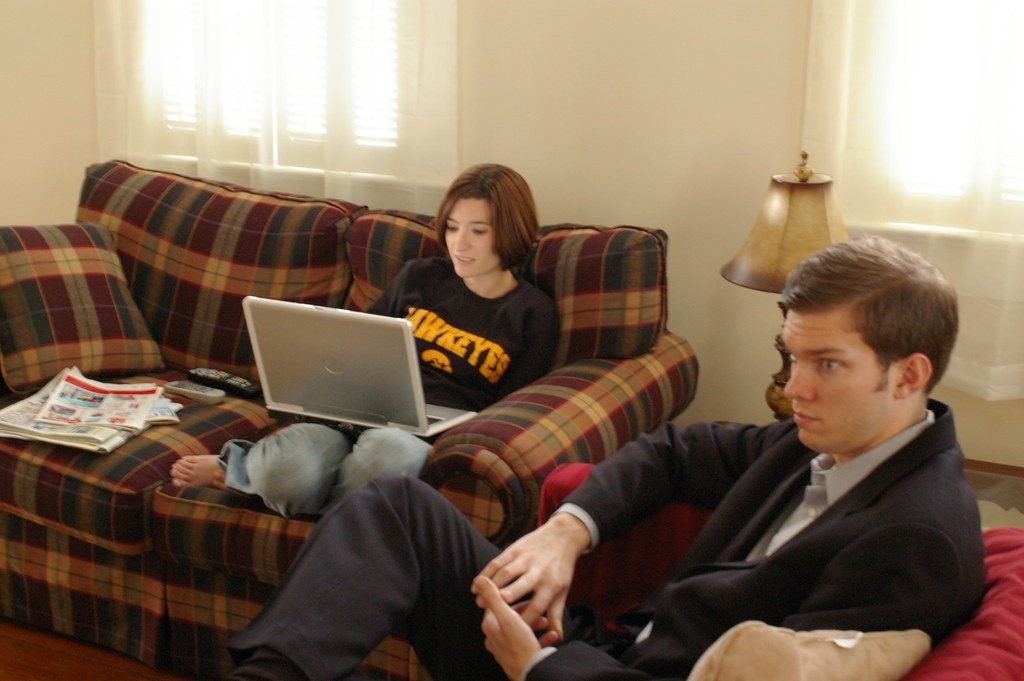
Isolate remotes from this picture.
[188,368,261,397]
[165,381,226,406]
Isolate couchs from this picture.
[0,159,699,681]
[540,462,1024,681]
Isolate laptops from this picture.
[242,295,478,437]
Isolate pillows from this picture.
[685,619,931,681]
[0,222,170,396]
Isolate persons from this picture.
[172,162,560,520]
[219,235,986,681]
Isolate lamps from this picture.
[719,151,851,424]
